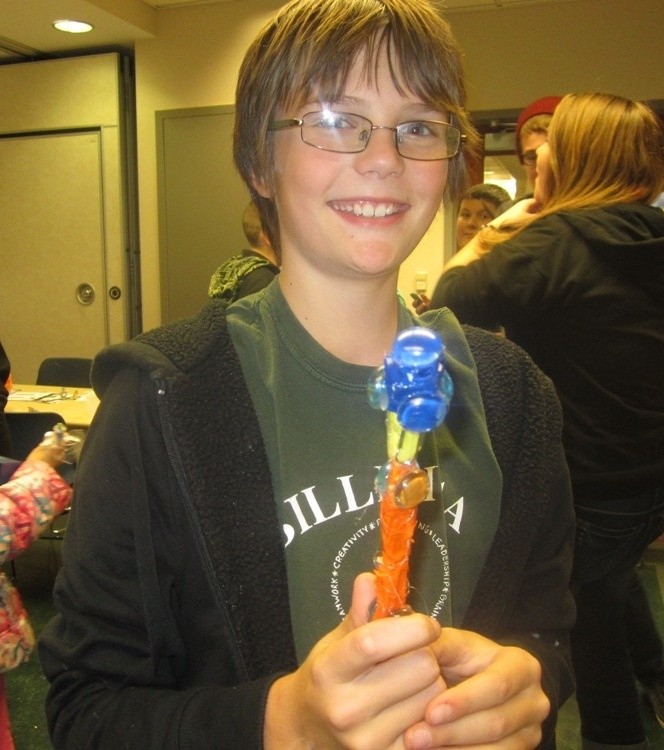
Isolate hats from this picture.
[514,95,564,164]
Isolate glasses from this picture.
[266,109,466,161]
[520,151,537,166]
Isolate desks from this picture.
[6,382,104,430]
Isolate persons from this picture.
[412,185,512,319]
[430,90,663,750]
[205,197,282,306]
[0,432,72,682]
[38,0,584,749]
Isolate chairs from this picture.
[0,410,83,578]
[38,358,94,390]
